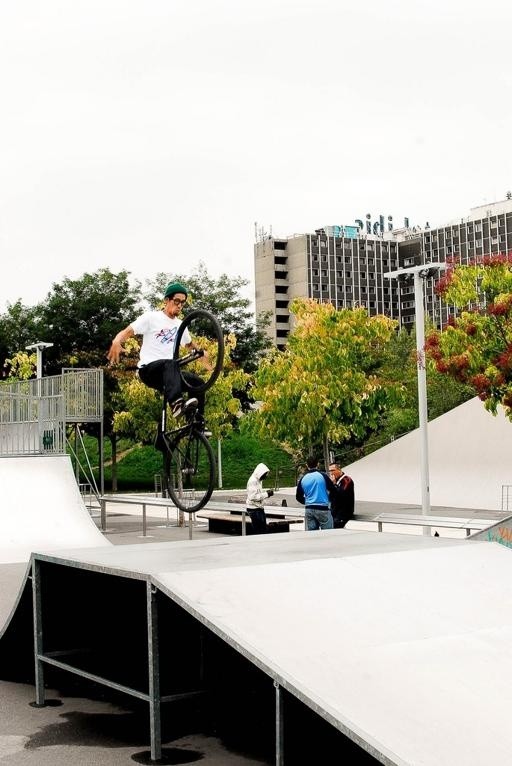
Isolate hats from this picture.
[164,282,187,297]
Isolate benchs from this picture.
[196,513,304,535]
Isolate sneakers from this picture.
[170,397,199,419]
[184,425,213,439]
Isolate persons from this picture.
[328,462,354,528]
[107,282,214,438]
[245,462,273,535]
[296,455,340,530]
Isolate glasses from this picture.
[171,298,187,307]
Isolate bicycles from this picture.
[107,308,225,512]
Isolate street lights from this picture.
[384,257,454,540]
[23,339,55,455]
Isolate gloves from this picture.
[267,490,273,497]
